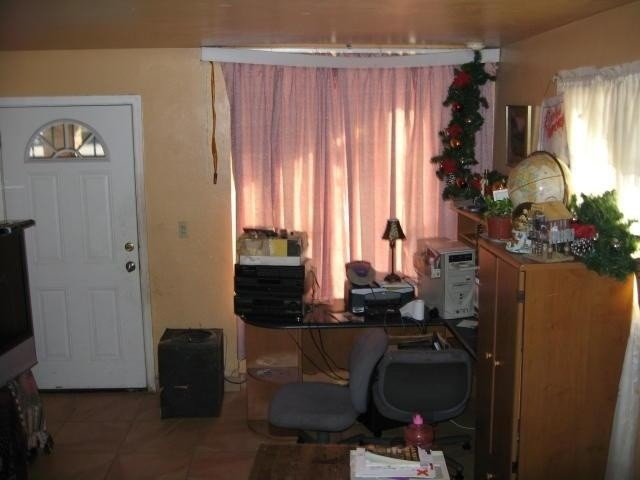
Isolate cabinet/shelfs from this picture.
[450,206,638,480]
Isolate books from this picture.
[349,443,451,480]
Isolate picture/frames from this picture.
[504,104,532,168]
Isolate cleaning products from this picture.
[402,415,433,449]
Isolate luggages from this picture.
[157,328,224,419]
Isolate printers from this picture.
[344,279,415,322]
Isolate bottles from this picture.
[404,415,434,455]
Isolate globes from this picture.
[505,150,572,254]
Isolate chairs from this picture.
[373,349,472,480]
[268,327,388,450]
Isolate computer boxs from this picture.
[416,236,476,320]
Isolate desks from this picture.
[249,444,356,480]
[241,299,460,440]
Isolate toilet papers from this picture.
[400,299,425,321]
